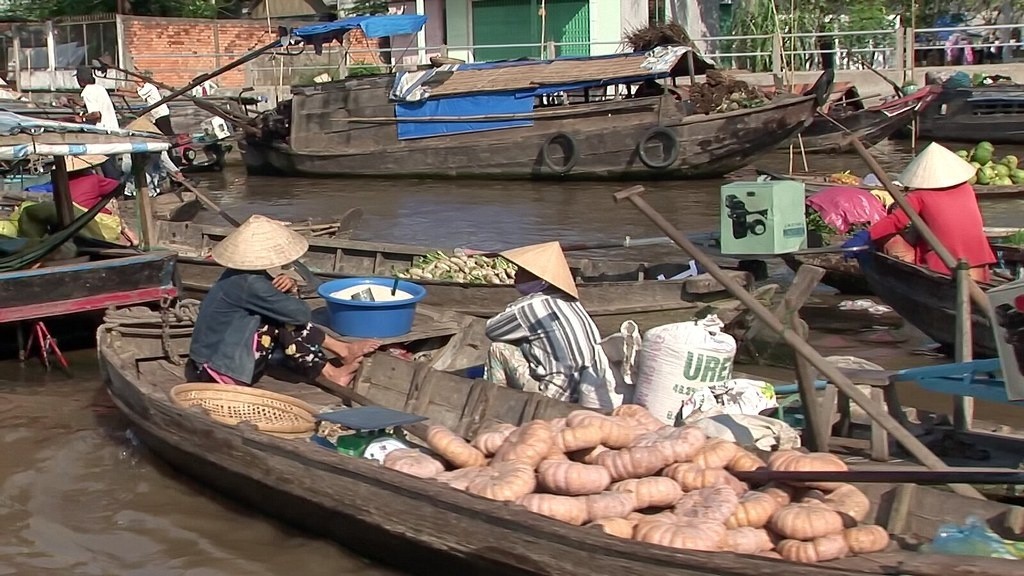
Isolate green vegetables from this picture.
[804,205,872,234]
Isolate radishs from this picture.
[395,251,518,285]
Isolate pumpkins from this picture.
[383,405,890,564]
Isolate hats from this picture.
[498,241,579,300]
[211,214,309,270]
[72,67,95,84]
[52,155,110,172]
[897,142,977,188]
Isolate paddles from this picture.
[178,177,320,286]
[836,129,1000,319]
[612,184,991,502]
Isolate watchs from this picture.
[82,114,86,123]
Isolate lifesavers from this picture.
[639,127,680,168]
[543,131,578,176]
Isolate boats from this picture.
[677,70,1024,155]
[0,95,233,342]
[95,302,1024,576]
[97,16,834,180]
[755,166,1024,231]
[779,228,1024,374]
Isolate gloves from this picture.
[840,231,872,258]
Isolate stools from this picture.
[816,365,911,461]
[312,408,429,458]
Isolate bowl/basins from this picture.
[317,277,427,338]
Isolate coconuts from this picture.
[955,141,1024,187]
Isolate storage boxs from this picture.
[721,181,808,253]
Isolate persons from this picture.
[122,116,182,196]
[60,155,134,246]
[137,80,172,136]
[839,143,997,281]
[69,66,122,196]
[485,239,612,403]
[185,214,383,389]
[191,81,217,99]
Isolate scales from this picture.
[312,403,432,466]
